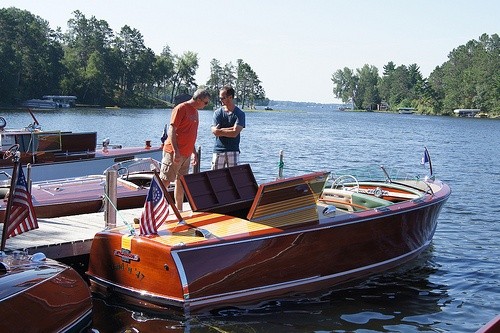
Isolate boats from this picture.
[0,250,95,333]
[43,95,77,108]
[264,107,273,110]
[0,156,176,223]
[81,144,451,319]
[0,106,167,184]
[23,98,60,111]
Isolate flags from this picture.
[138,174,169,237]
[421,147,429,165]
[6,161,39,240]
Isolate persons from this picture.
[158,88,210,214]
[210,86,245,170]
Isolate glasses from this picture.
[200,98,208,105]
[220,94,231,101]
